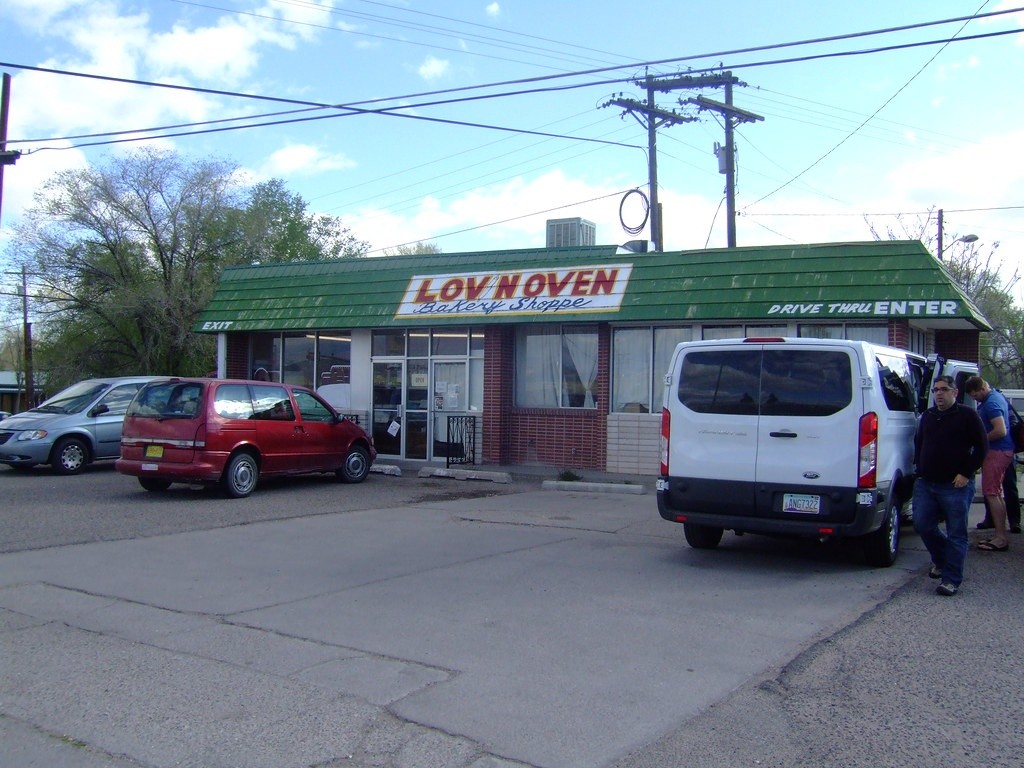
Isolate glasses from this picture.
[934,386,953,392]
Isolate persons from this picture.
[912,376,989,596]
[965,376,1022,550]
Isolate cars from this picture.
[0,411,12,423]
[115,375,378,498]
[0,376,177,476]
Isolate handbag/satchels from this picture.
[1001,394,1024,454]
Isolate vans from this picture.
[656,335,980,561]
[999,389,1024,421]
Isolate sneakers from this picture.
[929,567,942,579]
[936,582,958,595]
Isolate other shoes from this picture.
[1010,522,1021,532]
[977,523,995,529]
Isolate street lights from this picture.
[936,208,977,261]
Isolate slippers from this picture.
[977,539,1009,551]
